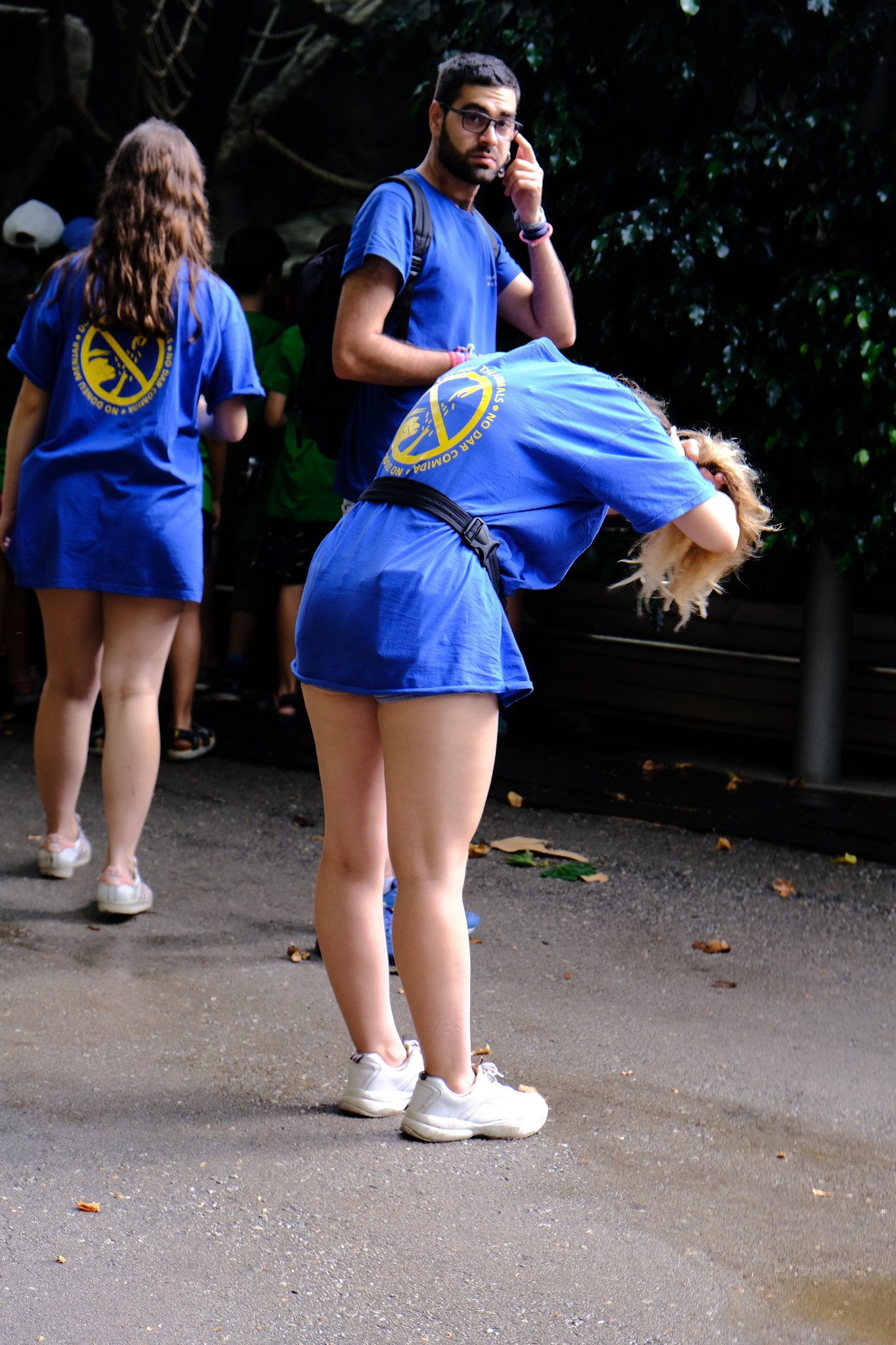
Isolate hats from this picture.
[4,197,65,250]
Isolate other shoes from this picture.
[383,878,479,935]
[316,904,400,973]
[38,813,93,878]
[96,856,154,914]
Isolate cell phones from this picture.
[506,139,521,172]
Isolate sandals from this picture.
[170,722,215,758]
[89,727,104,753]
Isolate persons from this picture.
[0,201,360,758]
[0,118,266,917]
[313,53,576,965]
[290,336,779,1146]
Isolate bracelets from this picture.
[511,209,553,246]
[447,344,485,370]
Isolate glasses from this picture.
[439,101,524,142]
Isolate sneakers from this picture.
[400,1057,548,1143]
[337,1034,425,1117]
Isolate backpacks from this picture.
[290,173,500,457]
